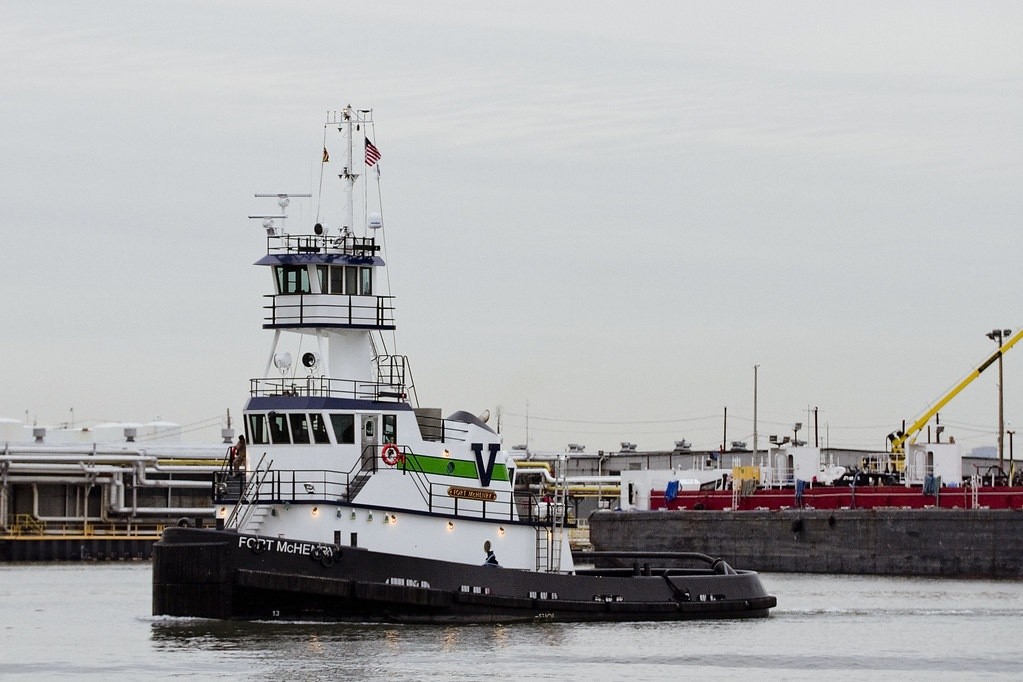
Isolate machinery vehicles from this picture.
[853,327,1023,487]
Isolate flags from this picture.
[322,148,330,162]
[364,136,382,167]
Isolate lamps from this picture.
[219,507,226,516]
[500,526,505,535]
[302,351,322,377]
[391,515,397,524]
[444,449,450,457]
[272,352,293,385]
[793,422,803,446]
[448,521,454,529]
[312,507,319,515]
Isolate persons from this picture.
[233,435,246,477]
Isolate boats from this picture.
[149,103,784,628]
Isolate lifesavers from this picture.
[381,444,401,466]
[232,447,240,460]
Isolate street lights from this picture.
[793,422,804,447]
[985,329,1013,475]
[768,435,792,451]
[1005,430,1015,471]
